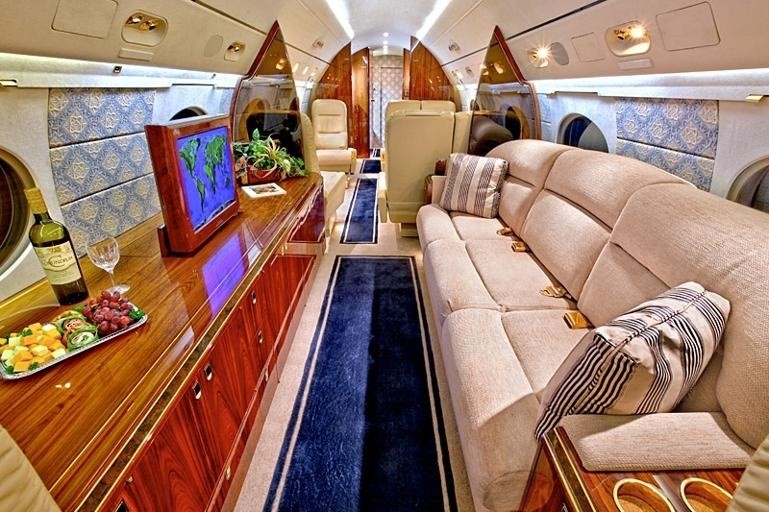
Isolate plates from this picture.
[0,301,148,380]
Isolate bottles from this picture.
[24,187,89,306]
[436,160,445,175]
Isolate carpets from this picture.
[373,149,380,157]
[262,254,458,512]
[340,178,378,243]
[359,160,381,173]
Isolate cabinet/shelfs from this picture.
[0,171,327,511]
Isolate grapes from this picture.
[87,290,133,334]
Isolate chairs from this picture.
[238,99,346,237]
[377,98,530,237]
[311,98,357,175]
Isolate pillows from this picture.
[533,279,732,444]
[438,152,509,220]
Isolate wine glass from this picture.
[85,236,130,295]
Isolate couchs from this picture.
[414,139,769,512]
